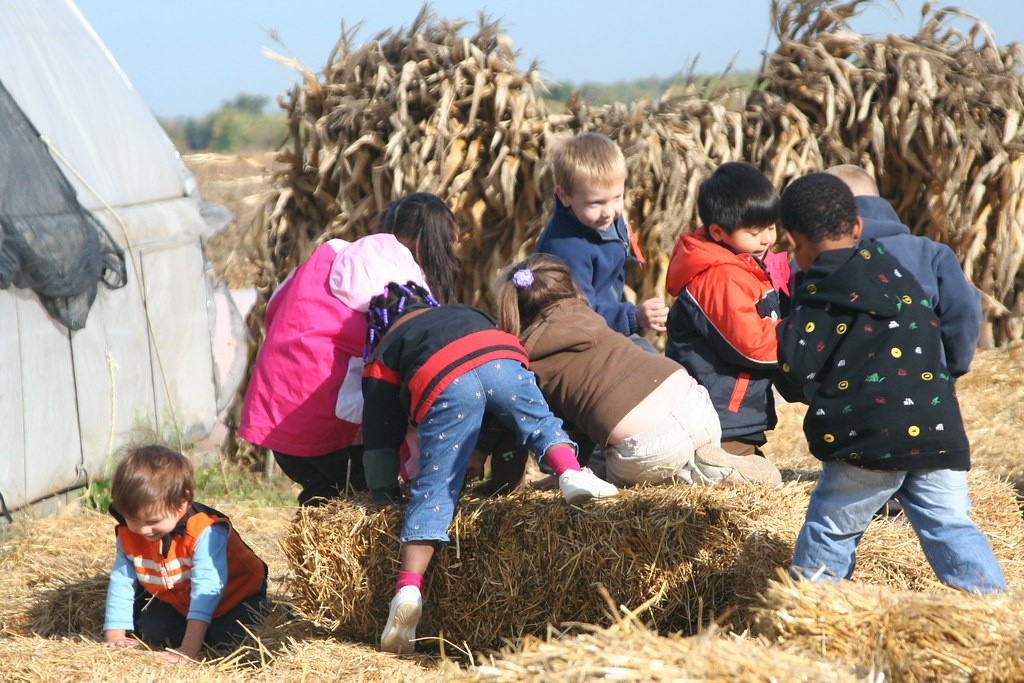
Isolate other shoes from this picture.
[297,486,331,507]
[696,444,782,486]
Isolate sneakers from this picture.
[380,585,423,654]
[558,465,619,505]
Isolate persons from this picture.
[240,193,462,507]
[102,445,296,665]
[531,132,669,352]
[665,161,1007,594]
[361,253,783,653]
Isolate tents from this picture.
[0,0,248,524]
